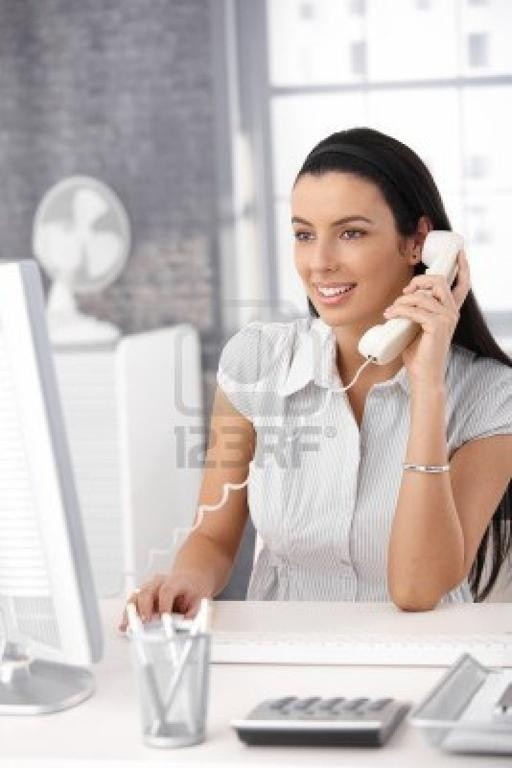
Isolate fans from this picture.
[32,174,133,348]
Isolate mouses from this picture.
[146,612,194,631]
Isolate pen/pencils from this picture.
[127,598,209,736]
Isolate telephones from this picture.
[358,230,465,366]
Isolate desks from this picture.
[0,606,511,768]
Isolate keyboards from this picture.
[206,633,512,668]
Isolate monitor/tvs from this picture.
[0,259,105,715]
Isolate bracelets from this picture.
[402,461,453,474]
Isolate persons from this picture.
[119,129,512,628]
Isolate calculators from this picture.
[232,696,412,746]
